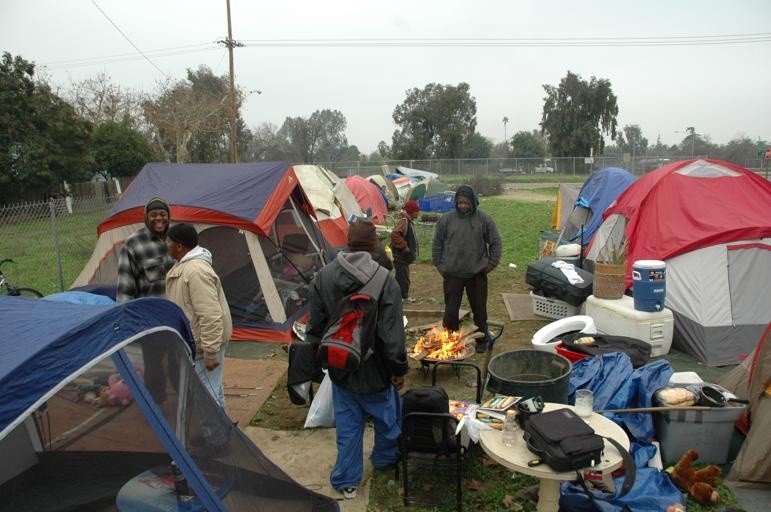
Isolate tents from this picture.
[0,294,341,512]
[291,164,364,249]
[340,175,388,225]
[583,159,771,367]
[556,166,636,245]
[68,160,339,345]
[712,322,770,484]
[365,166,439,212]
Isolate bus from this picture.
[528,158,557,176]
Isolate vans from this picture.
[638,158,672,171]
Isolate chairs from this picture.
[395,412,466,511]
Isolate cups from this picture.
[573,388,595,427]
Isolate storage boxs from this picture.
[586,295,674,358]
[653,383,745,465]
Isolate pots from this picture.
[696,385,753,408]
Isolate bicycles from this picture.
[0,254,44,299]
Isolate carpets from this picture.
[37,357,289,452]
[501,293,552,321]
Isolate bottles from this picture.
[500,408,520,457]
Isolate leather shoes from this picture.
[477,341,487,352]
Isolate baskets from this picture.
[530,290,578,318]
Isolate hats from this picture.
[347,220,378,250]
[147,202,167,212]
[406,201,419,214]
[168,223,199,249]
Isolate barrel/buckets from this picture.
[631,258,667,314]
[485,349,573,406]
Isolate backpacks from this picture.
[402,386,450,453]
[317,265,390,372]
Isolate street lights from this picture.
[237,88,266,103]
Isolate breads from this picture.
[658,386,696,407]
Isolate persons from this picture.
[307,219,409,499]
[165,222,233,457]
[116,196,177,406]
[431,185,502,354]
[390,200,420,303]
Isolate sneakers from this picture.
[402,296,416,304]
[342,485,359,499]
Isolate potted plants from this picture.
[593,237,629,298]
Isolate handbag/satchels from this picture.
[523,408,605,472]
[526,255,595,307]
